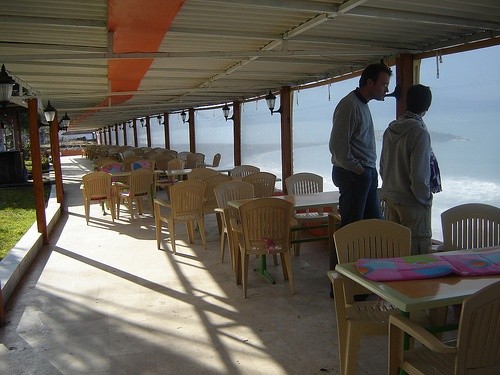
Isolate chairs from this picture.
[80,146,500,375]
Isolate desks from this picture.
[108,170,164,208]
[228,188,382,284]
[336,245,500,375]
[165,167,234,181]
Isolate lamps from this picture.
[58,113,71,132]
[38,100,56,129]
[0,64,16,108]
[140,119,146,128]
[180,110,190,123]
[157,115,165,125]
[222,105,234,121]
[93,120,135,135]
[265,89,282,115]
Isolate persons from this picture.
[380,83,441,255]
[329,62,392,302]
